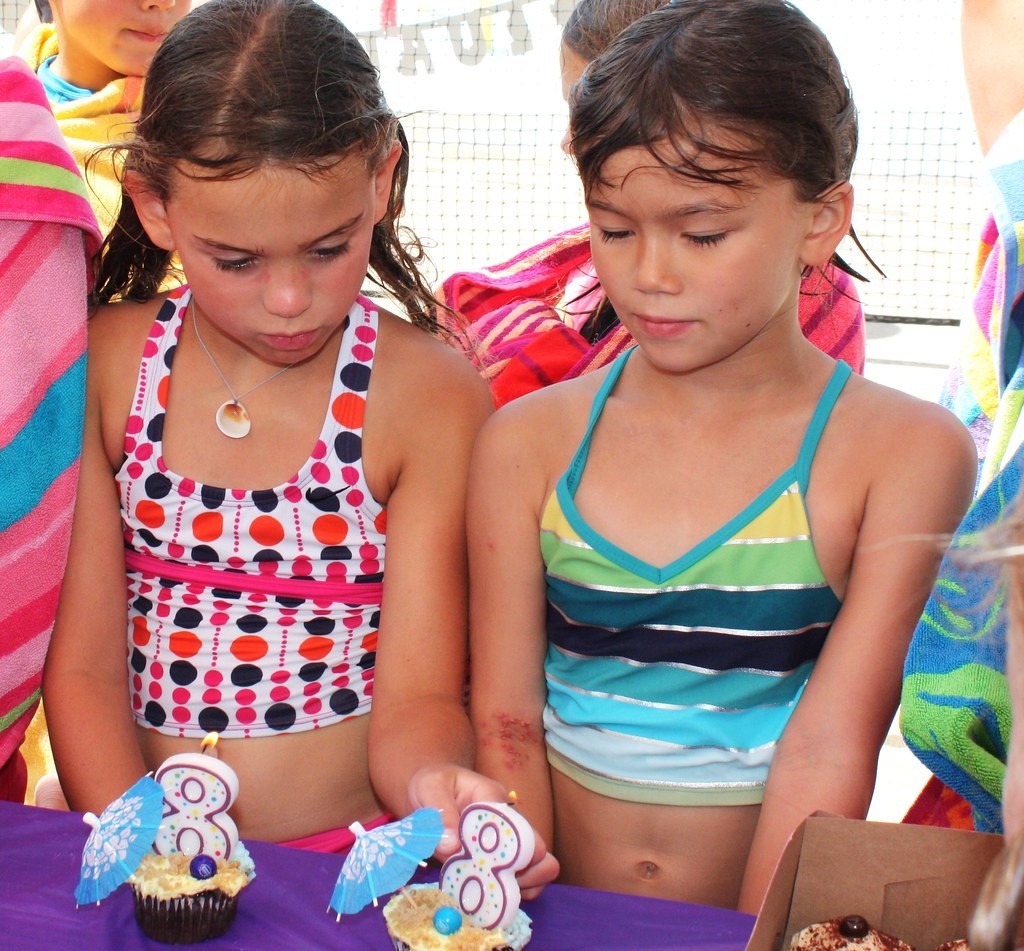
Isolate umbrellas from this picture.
[325,806,444,926]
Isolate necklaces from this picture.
[187,296,298,440]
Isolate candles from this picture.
[437,790,537,931]
[146,730,240,865]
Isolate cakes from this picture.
[385,886,514,951]
[790,917,913,951]
[130,852,243,943]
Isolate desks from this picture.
[0,799,759,951]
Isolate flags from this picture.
[75,770,171,905]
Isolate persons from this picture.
[432,0,888,422]
[22,0,198,294]
[902,0,1023,846]
[461,0,977,919]
[42,0,563,904]
